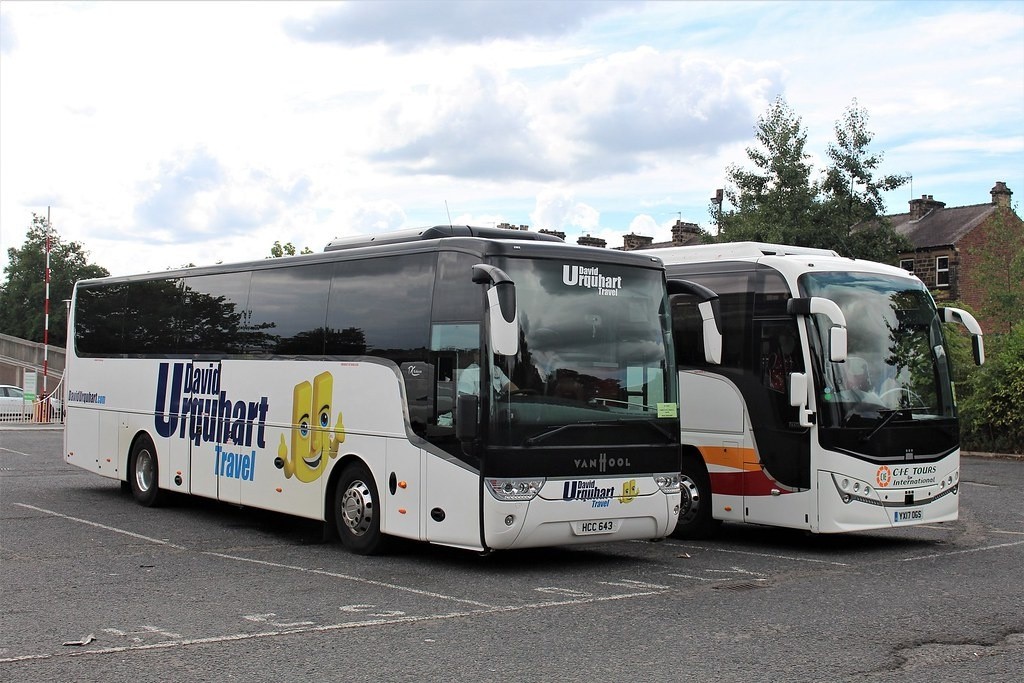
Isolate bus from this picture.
[64,224,722,556]
[625,242,986,540]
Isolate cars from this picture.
[0,384,62,418]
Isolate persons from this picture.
[456,347,523,423]
